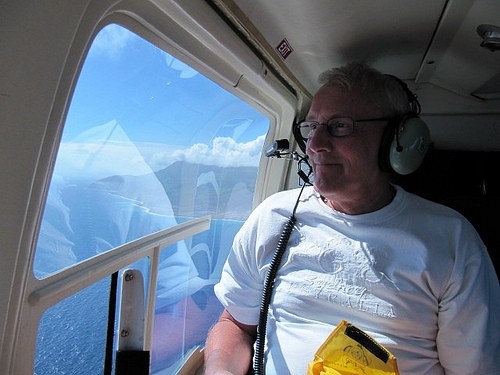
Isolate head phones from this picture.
[293,74,431,177]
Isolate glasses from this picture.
[296,116,390,139]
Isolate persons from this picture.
[204,63,500,375]
[32,24,209,375]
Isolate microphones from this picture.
[265,139,308,164]
